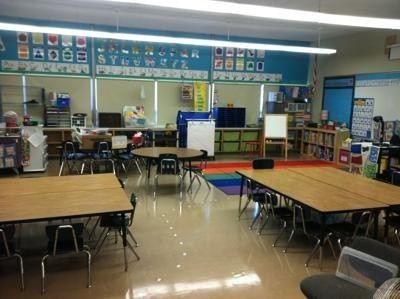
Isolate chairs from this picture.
[0,128,211,295]
[238,157,400,270]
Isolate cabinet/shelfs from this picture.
[215,100,372,174]
[45,92,86,127]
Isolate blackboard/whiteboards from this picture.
[264,114,288,138]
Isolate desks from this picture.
[365,146,400,179]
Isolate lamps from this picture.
[108,1,400,33]
[0,1,338,56]
[373,115,390,146]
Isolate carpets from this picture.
[184,159,349,195]
